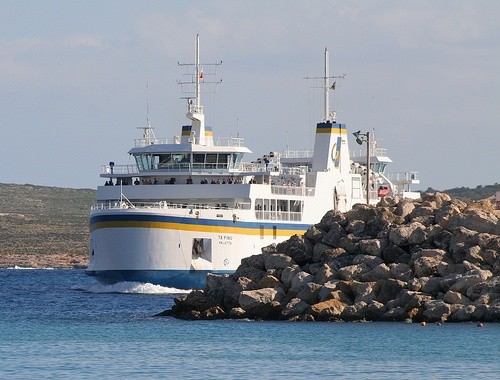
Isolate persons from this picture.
[102,175,260,186]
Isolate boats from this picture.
[89,33,417,290]
